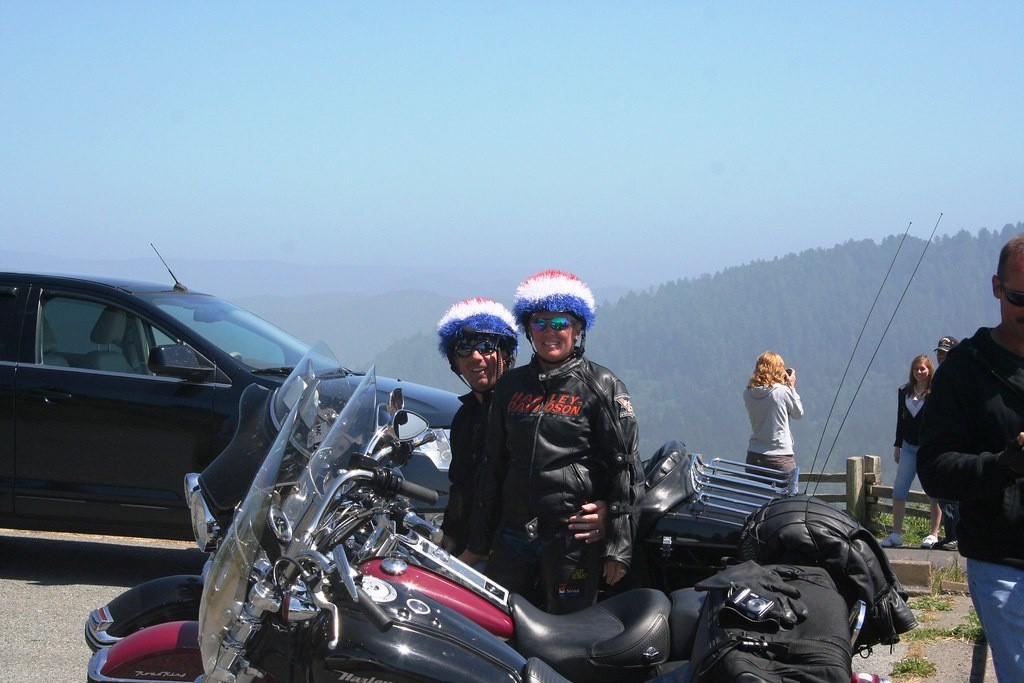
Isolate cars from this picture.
[0,271,463,556]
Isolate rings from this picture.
[596,529,600,537]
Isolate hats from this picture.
[934,336,959,352]
[436,297,522,375]
[512,270,597,334]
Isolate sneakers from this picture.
[921,535,939,548]
[881,533,903,547]
[932,539,959,550]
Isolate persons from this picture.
[879,355,945,548]
[436,295,610,557]
[744,351,805,488]
[932,335,960,551]
[915,236,1024,683]
[454,270,646,617]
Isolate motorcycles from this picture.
[85,336,798,683]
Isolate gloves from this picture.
[694,560,808,634]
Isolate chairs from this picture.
[42,317,69,367]
[75,306,135,373]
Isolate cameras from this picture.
[782,369,793,381]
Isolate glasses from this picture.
[529,316,576,332]
[455,340,496,358]
[997,273,1024,307]
